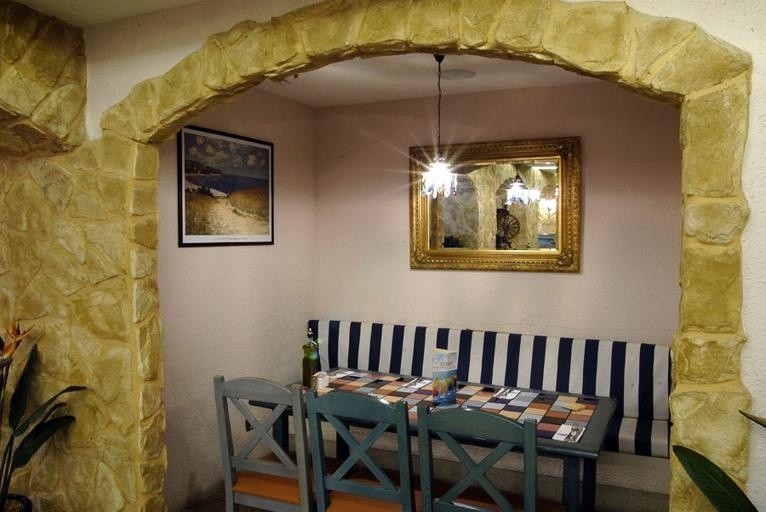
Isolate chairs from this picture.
[304,388,421,512]
[213,375,331,511]
[417,401,538,511]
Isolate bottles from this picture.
[300,327,318,387]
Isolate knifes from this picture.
[572,423,583,443]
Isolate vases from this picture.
[6,493,33,512]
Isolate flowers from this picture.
[0,320,87,512]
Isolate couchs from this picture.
[308,319,669,509]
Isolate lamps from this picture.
[421,53,458,199]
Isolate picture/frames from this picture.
[408,134,584,274]
[173,121,274,246]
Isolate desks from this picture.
[249,365,618,510]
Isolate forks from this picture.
[565,421,577,440]
